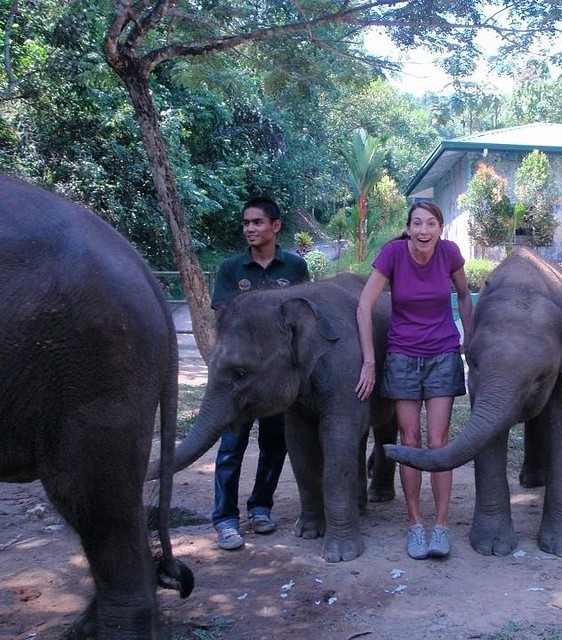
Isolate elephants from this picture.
[0,170,194,640]
[146,273,397,563]
[380,245,562,556]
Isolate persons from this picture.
[211,198,310,552]
[354,203,475,559]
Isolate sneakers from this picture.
[217,528,244,549]
[427,528,450,555]
[250,514,276,532]
[407,528,428,560]
